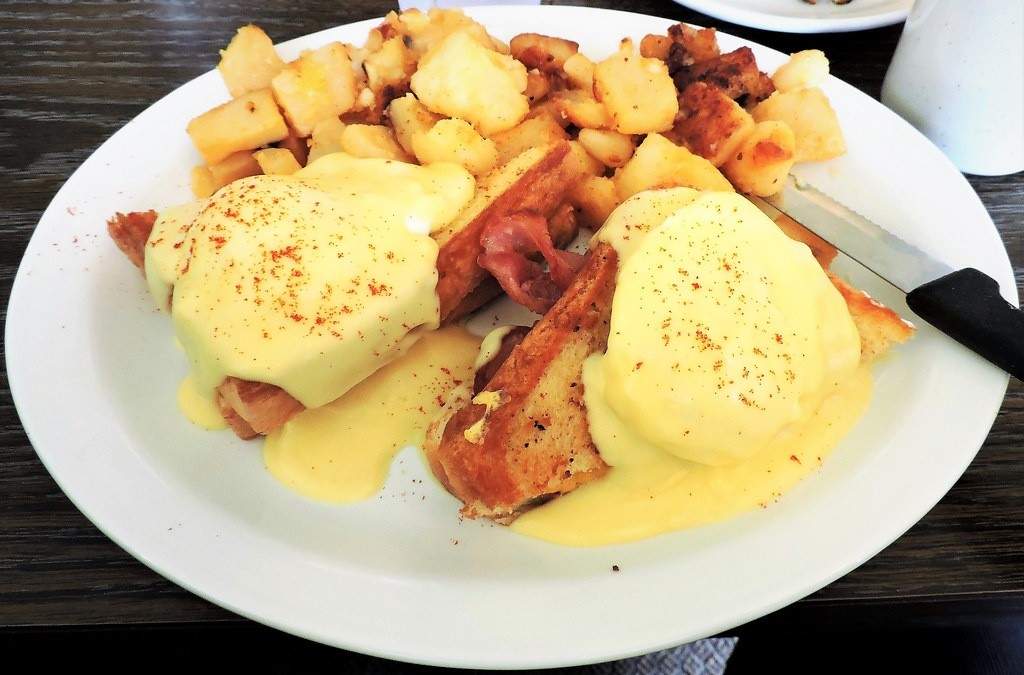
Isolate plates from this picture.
[673,0,916,33]
[5,5,1019,670]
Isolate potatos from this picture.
[185,4,848,230]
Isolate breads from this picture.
[104,138,577,438]
[438,182,914,516]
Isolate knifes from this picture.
[757,174,1024,383]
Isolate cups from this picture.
[881,0,1024,176]
